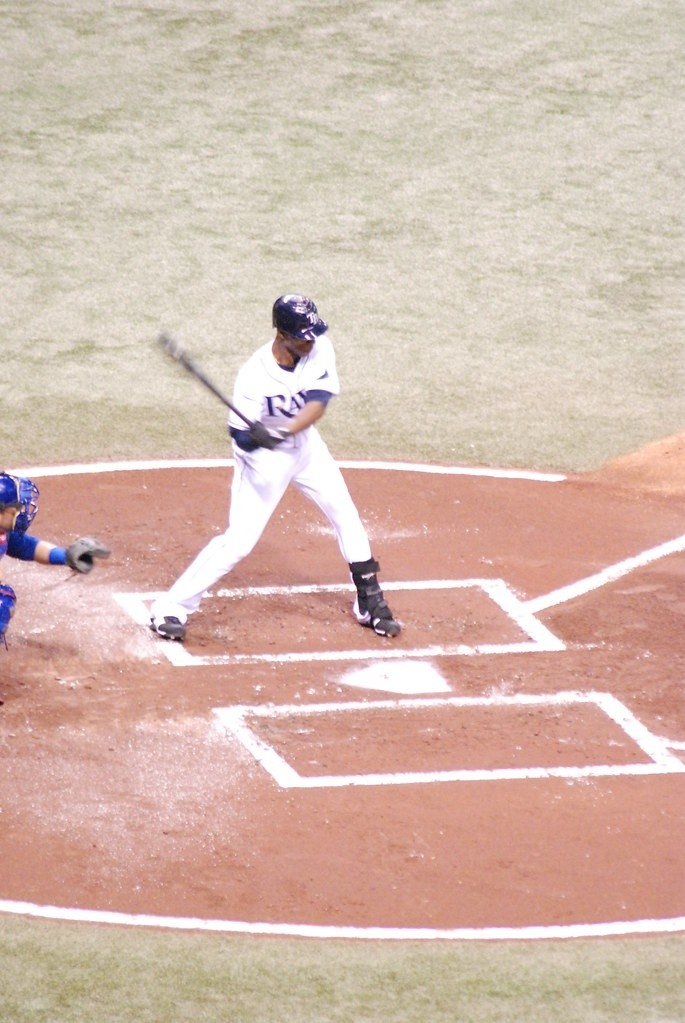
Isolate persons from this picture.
[149,294,401,639]
[0,473,111,642]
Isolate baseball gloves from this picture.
[65,534,111,574]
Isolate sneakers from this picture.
[148,601,186,640]
[353,592,401,638]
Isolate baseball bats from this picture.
[157,331,255,428]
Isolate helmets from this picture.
[0,473,39,534]
[272,293,329,341]
